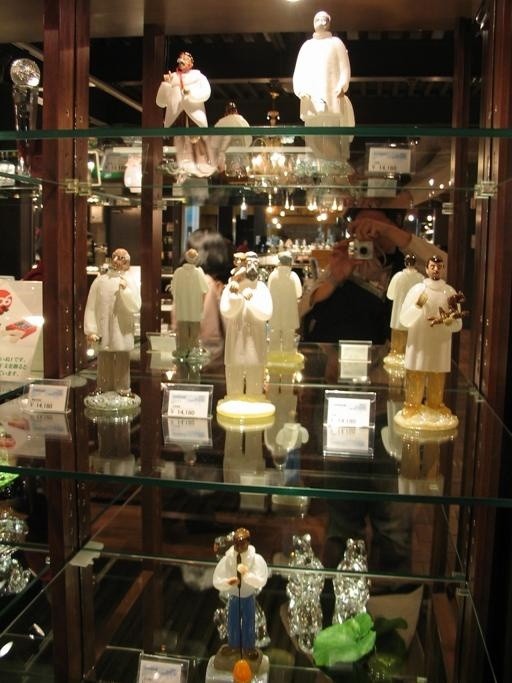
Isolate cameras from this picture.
[348,240,374,261]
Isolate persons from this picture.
[91,419,136,476]
[400,254,462,418]
[225,371,295,477]
[267,252,302,359]
[380,381,443,497]
[212,527,267,660]
[173,359,201,464]
[155,10,355,185]
[84,249,141,399]
[299,190,448,594]
[387,254,425,358]
[171,228,236,375]
[221,252,272,401]
[172,249,209,354]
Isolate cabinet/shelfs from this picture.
[0,1,511,681]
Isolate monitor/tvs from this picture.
[88,151,102,190]
